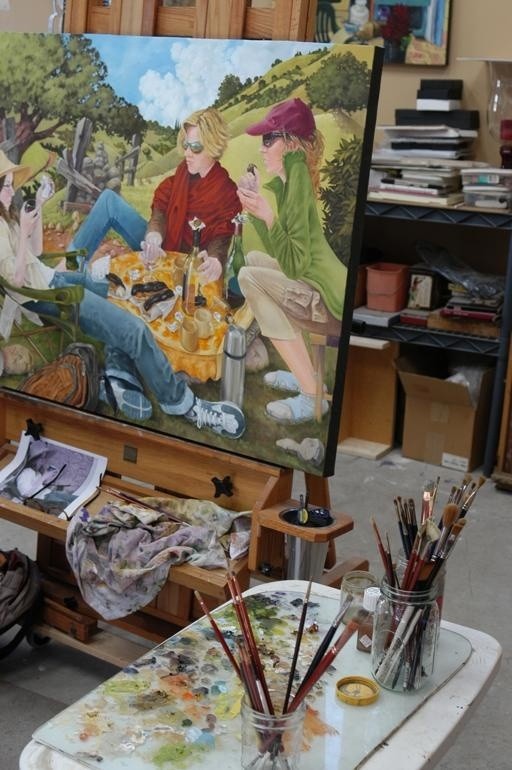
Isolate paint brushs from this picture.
[370,474,485,692]
[96,486,192,527]
[194,558,369,770]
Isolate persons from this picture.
[14,441,76,514]
[57,107,241,286]
[0,145,247,440]
[239,98,347,423]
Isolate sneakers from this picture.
[182,395,246,441]
[99,374,154,422]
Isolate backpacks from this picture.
[16,341,99,414]
[0,547,46,662]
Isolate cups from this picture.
[193,309,216,342]
[280,506,337,585]
[181,316,200,353]
[144,240,160,272]
[239,690,304,770]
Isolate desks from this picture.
[15,578,506,770]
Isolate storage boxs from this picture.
[390,348,496,475]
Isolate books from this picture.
[365,125,490,210]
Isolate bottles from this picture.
[221,223,246,306]
[219,325,248,411]
[183,230,207,316]
[340,547,445,696]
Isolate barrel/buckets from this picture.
[278,507,332,582]
[364,260,411,313]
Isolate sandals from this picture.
[264,396,330,426]
[266,369,326,393]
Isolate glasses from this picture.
[262,131,290,147]
[183,142,204,154]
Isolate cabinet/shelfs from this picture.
[349,191,512,480]
[0,390,353,671]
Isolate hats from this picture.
[0,150,32,190]
[246,98,316,136]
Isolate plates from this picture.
[112,295,129,301]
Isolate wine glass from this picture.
[236,179,260,224]
[22,199,40,240]
